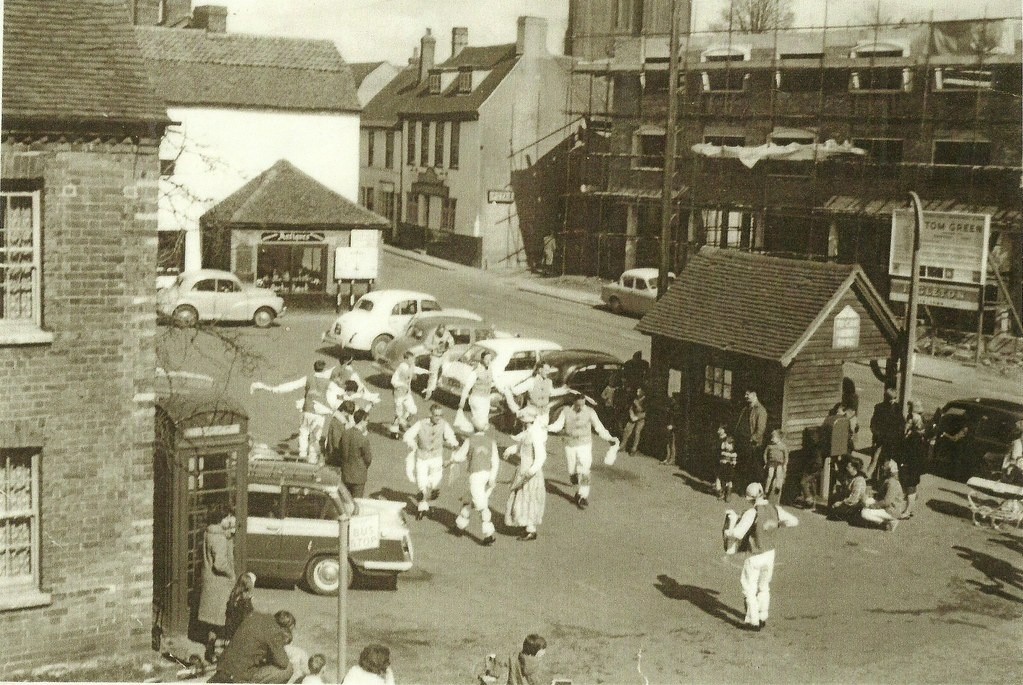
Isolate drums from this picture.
[723,512,757,552]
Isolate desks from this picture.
[966,476,1023,531]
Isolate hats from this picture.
[745,482,764,501]
[517,406,537,422]
[472,414,489,432]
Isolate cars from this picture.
[926,395,1023,478]
[600,268,678,318]
[320,289,627,436]
[156,269,285,330]
[246,456,416,597]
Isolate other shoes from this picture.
[432,489,438,500]
[447,525,464,537]
[725,486,730,502]
[415,511,426,520]
[575,492,589,510]
[480,536,495,545]
[744,620,765,633]
[898,512,913,520]
[715,489,724,499]
[630,450,636,457]
[391,430,400,439]
[618,447,624,453]
[514,530,537,542]
[570,475,578,484]
[660,459,674,466]
[888,519,899,532]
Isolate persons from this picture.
[225,571,256,641]
[478,634,547,685]
[800,378,926,532]
[601,350,648,456]
[296,654,326,684]
[197,501,238,664]
[402,402,460,521]
[424,323,454,402]
[541,232,556,265]
[503,395,620,541]
[940,407,972,456]
[1001,431,1023,486]
[510,359,597,430]
[390,346,435,438]
[442,413,500,545]
[343,644,395,685]
[724,482,800,631]
[454,350,505,432]
[714,382,789,502]
[250,354,381,496]
[206,610,296,685]
[660,397,679,466]
[256,266,322,293]
